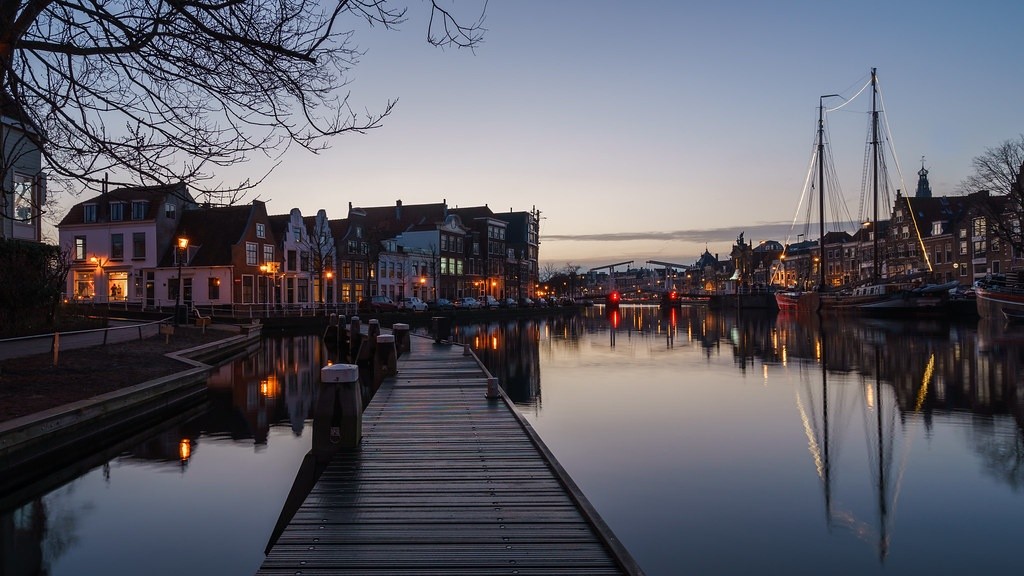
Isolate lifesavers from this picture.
[851,281,856,288]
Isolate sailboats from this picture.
[765,63,1024,328]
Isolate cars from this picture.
[358,295,399,314]
[438,298,459,311]
[398,297,428,312]
[477,295,576,311]
[455,296,482,311]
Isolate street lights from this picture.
[420,278,425,300]
[174,232,190,305]
[259,264,268,318]
[325,271,334,303]
